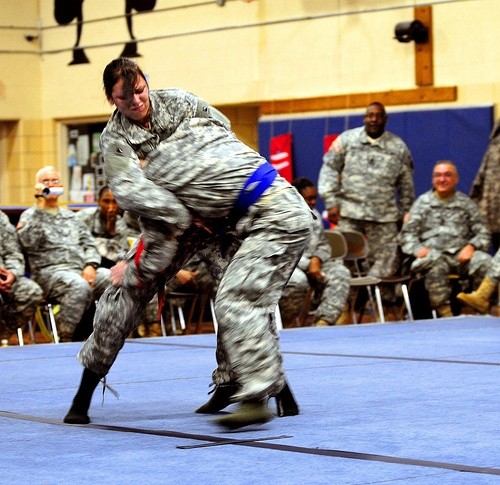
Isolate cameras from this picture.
[34,182,65,200]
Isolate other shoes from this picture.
[148,323,161,335]
[313,308,350,326]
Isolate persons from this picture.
[108,115,313,430]
[65,55,300,426]
[0,93,500,350]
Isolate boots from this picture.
[457,275,496,313]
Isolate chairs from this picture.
[324,231,466,324]
[16,263,62,344]
[159,287,216,337]
